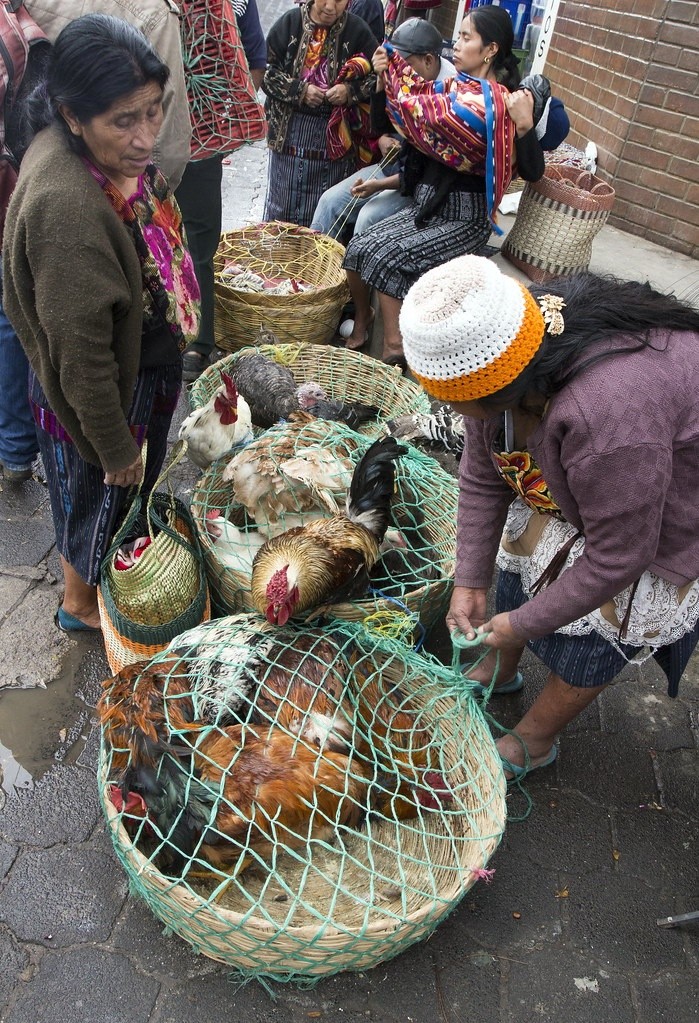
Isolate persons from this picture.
[411,71,571,173]
[262,0,384,240]
[398,254,699,780]
[309,17,461,350]
[340,5,546,377]
[0,0,267,633]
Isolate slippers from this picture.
[493,738,557,784]
[443,662,523,697]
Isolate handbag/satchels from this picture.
[109,438,200,625]
[500,164,616,285]
[96,491,212,676]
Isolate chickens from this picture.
[207,507,267,577]
[228,411,421,537]
[228,353,388,430]
[94,611,450,877]
[250,436,413,626]
[178,369,255,465]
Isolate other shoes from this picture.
[182,351,202,372]
[0,459,32,483]
[58,607,102,631]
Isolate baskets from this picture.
[97,612,508,973]
[209,222,354,352]
[192,341,432,442]
[188,422,461,636]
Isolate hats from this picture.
[399,253,546,402]
[389,17,443,60]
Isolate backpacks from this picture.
[0,0,54,229]
